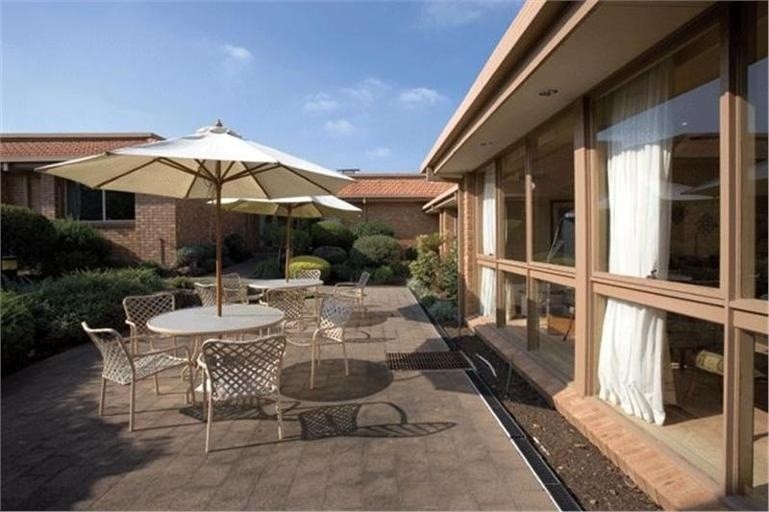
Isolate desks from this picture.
[144,303,286,416]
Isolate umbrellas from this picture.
[204,194,364,282]
[32,118,365,339]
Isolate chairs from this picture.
[194,268,321,315]
[258,283,320,338]
[332,271,372,318]
[280,294,357,392]
[123,291,175,383]
[196,334,287,456]
[81,321,195,433]
